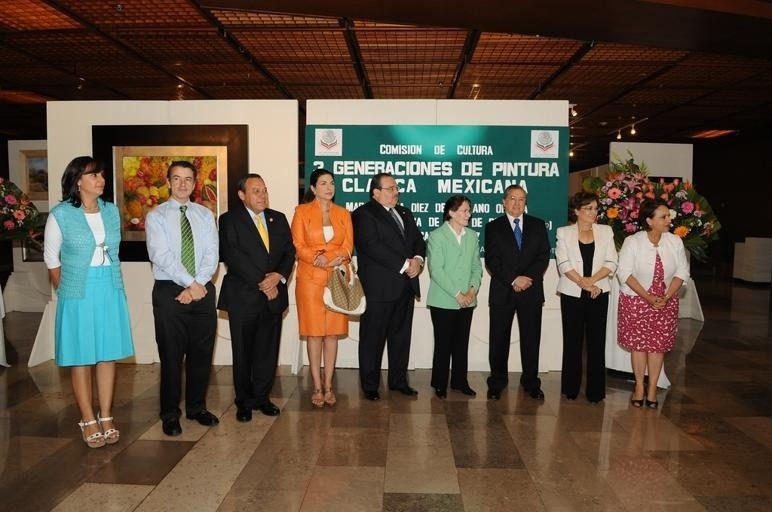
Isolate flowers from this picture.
[0,176,48,241]
[567,147,720,263]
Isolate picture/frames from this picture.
[19,149,49,201]
[116,145,228,242]
[22,212,49,262]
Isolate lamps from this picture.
[569,103,579,117]
[606,116,649,139]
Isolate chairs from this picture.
[731,236,772,286]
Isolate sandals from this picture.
[646,397,658,408]
[632,399,644,407]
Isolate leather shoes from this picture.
[435,387,447,399]
[186,408,219,426]
[237,407,252,421]
[525,385,544,400]
[253,401,280,415]
[487,386,501,399]
[365,391,380,400]
[451,382,476,396]
[389,382,417,395]
[163,418,182,436]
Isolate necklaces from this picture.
[579,229,592,232]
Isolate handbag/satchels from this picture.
[323,265,366,315]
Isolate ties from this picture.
[513,219,522,250]
[180,206,195,278]
[255,215,269,253]
[389,208,404,237]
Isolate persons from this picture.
[291,168,355,406]
[216,173,296,422]
[426,195,483,399]
[555,192,618,404]
[350,173,428,401]
[43,156,135,449]
[616,197,692,408]
[144,161,219,436]
[483,185,551,400]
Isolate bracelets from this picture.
[664,294,671,300]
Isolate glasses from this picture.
[582,205,598,212]
[657,214,671,219]
[382,186,399,191]
[506,196,524,202]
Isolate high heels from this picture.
[324,388,336,405]
[312,389,324,408]
[97,409,120,444]
[78,419,107,449]
